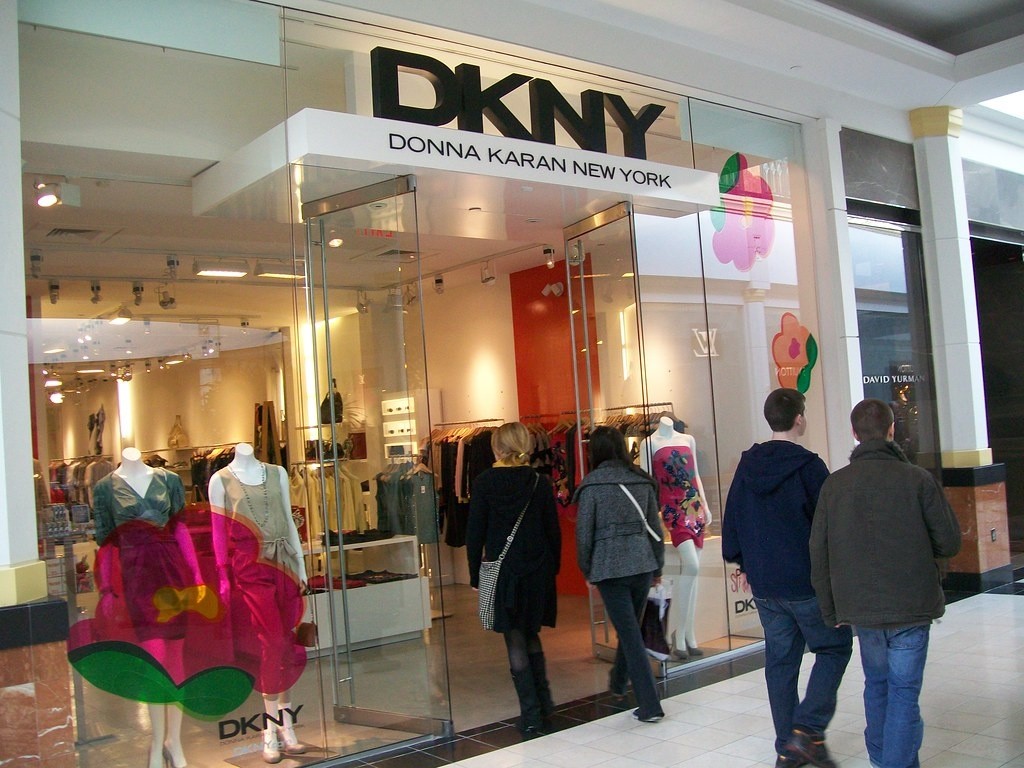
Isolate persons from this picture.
[571,426,664,722]
[809,397,962,768]
[722,388,854,768]
[208,442,308,764]
[466,422,560,742]
[640,416,713,660]
[92,447,206,768]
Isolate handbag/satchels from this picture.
[167,414,188,448]
[477,560,501,631]
[306,434,344,459]
[641,582,672,661]
[321,378,342,423]
[292,579,318,647]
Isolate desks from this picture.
[39,530,96,624]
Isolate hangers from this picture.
[50,456,107,466]
[292,458,352,478]
[371,456,440,480]
[521,402,690,439]
[192,447,236,460]
[424,424,498,439]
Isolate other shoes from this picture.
[609,668,626,702]
[514,716,542,734]
[542,708,554,718]
[632,708,663,722]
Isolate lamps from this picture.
[478,258,498,287]
[163,256,181,280]
[107,307,134,326]
[30,175,83,209]
[48,280,60,304]
[158,284,180,311]
[569,243,586,266]
[239,319,252,337]
[540,281,564,298]
[541,241,559,272]
[191,256,251,278]
[89,282,103,304]
[402,286,418,307]
[353,290,377,315]
[29,250,45,279]
[254,258,305,280]
[432,272,449,295]
[132,282,145,306]
[382,288,408,315]
[44,317,219,409]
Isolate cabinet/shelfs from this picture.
[295,530,435,664]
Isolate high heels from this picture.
[276,725,306,754]
[671,629,688,659]
[685,637,703,656]
[261,728,280,763]
[163,742,188,768]
[147,746,151,768]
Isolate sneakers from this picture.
[774,753,810,768]
[783,724,838,768]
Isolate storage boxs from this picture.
[40,553,83,576]
[48,573,79,597]
[72,503,92,523]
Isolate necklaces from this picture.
[228,461,269,528]
